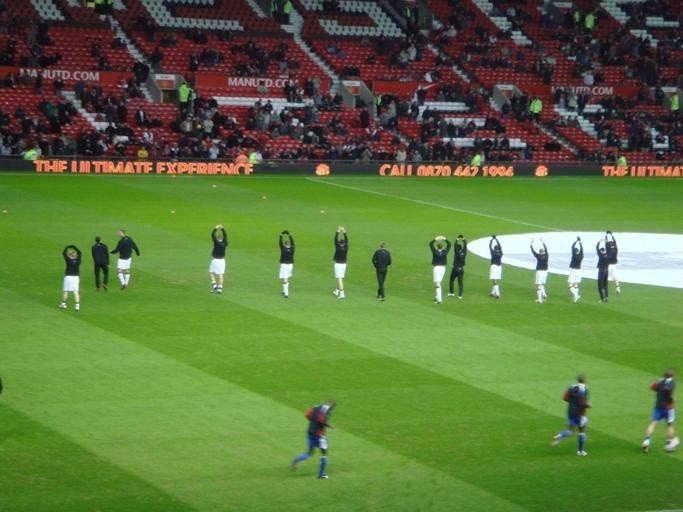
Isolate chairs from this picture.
[0,1,681,159]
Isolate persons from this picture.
[332,226,349,299]
[1,0,682,161]
[276,230,296,300]
[289,399,334,479]
[428,231,623,303]
[375,242,391,303]
[640,368,679,455]
[208,225,229,296]
[59,228,140,313]
[550,372,594,457]
